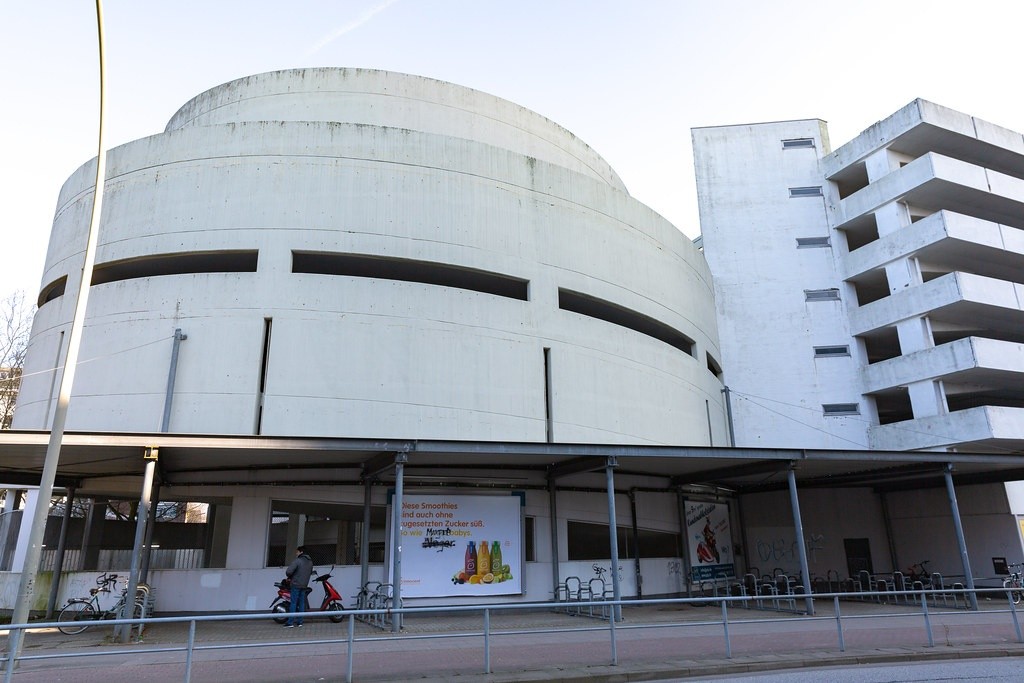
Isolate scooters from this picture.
[270,565,345,624]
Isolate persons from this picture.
[283,546,313,628]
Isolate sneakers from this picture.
[284,624,303,628]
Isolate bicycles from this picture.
[56,572,143,635]
[1003,562,1024,604]
[904,560,933,587]
